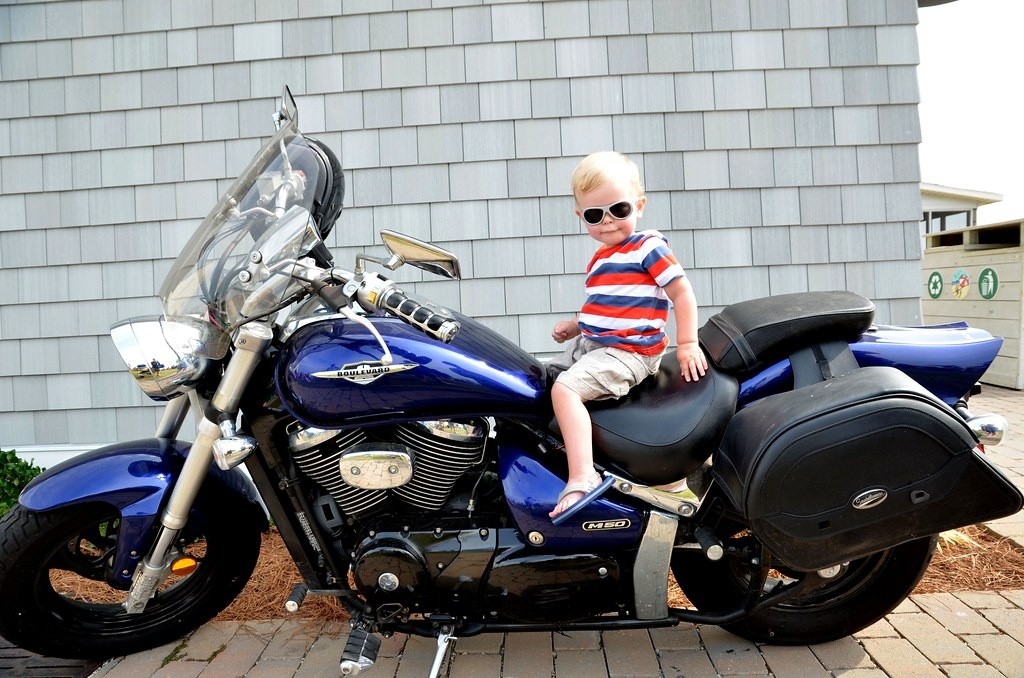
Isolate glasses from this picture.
[581,196,642,226]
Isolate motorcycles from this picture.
[0,85,1024,678]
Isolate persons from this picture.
[548,151,708,525]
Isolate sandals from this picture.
[550,471,616,526]
